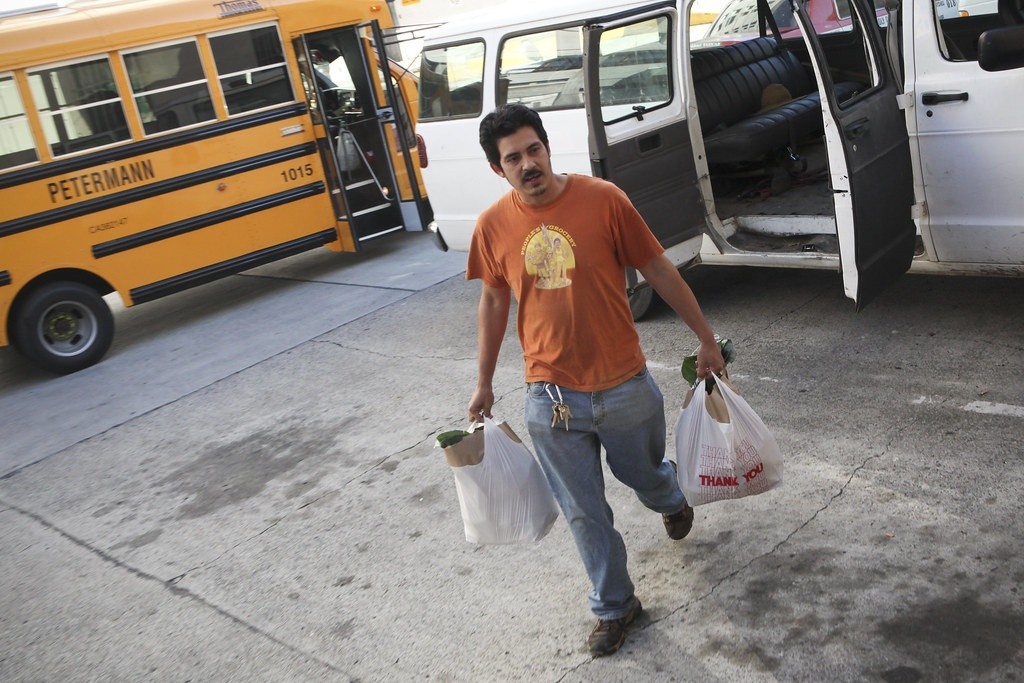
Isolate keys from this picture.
[551,402,573,431]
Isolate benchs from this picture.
[686,37,869,177]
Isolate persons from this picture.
[465,104,725,657]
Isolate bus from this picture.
[0,1,451,376]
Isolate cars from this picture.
[507,0,997,75]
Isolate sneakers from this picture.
[663,459,696,542]
[587,599,643,656]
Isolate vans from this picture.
[408,0,1024,318]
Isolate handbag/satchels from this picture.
[674,369,784,506]
[450,410,560,546]
